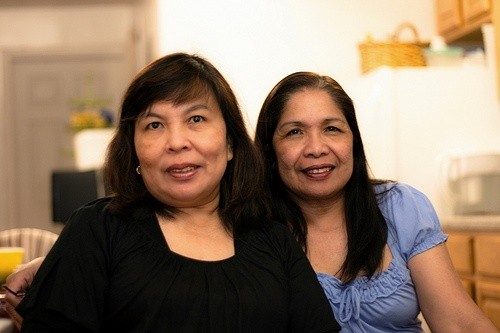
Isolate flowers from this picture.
[67,102,115,132]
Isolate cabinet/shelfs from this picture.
[419,212,500,333]
[433,1,497,45]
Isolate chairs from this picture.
[1,228,62,319]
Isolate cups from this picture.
[0,246,25,284]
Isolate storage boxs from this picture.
[359,21,430,73]
